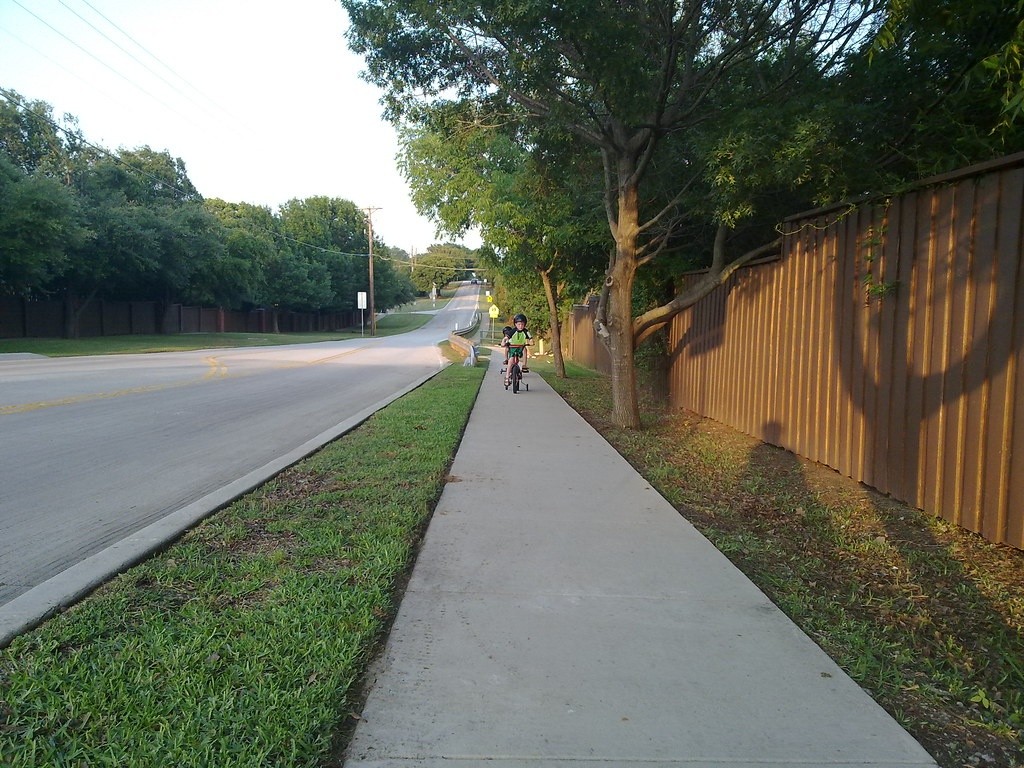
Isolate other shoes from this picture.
[503,360,508,364]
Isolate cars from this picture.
[471,279,478,285]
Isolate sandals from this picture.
[504,377,509,386]
[523,365,529,369]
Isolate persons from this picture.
[503,326,513,364]
[501,314,534,386]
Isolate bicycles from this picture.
[498,340,535,395]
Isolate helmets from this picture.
[514,314,527,322]
[503,327,512,334]
